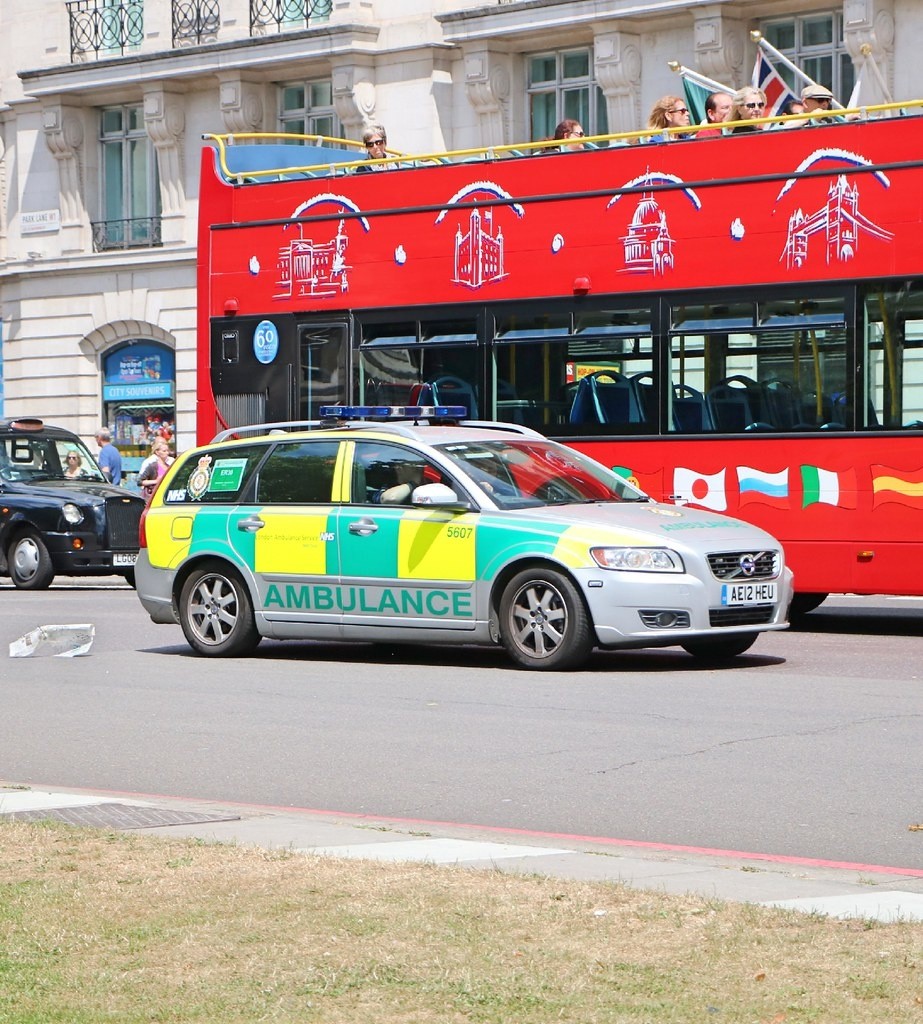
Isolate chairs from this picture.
[227,109,918,189]
[408,371,883,434]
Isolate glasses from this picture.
[68,456,77,461]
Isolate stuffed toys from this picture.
[146,416,172,442]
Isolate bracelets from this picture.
[408,481,417,489]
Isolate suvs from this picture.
[0,417,149,590]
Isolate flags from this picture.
[752,48,796,130]
[682,78,716,126]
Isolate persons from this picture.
[696,92,734,138]
[647,95,694,142]
[95,427,121,486]
[726,87,767,134]
[772,99,804,130]
[62,450,87,476]
[451,450,522,503]
[356,124,398,173]
[540,119,585,153]
[140,436,176,474]
[0,443,44,470]
[137,442,172,498]
[785,84,860,129]
[371,459,432,506]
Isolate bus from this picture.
[196,97,922,629]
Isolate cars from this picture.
[133,405,797,673]
